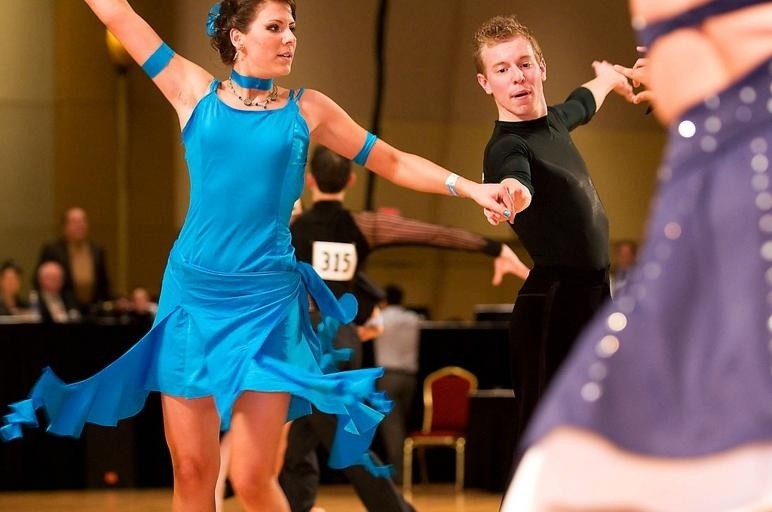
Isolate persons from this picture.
[373,283,421,485]
[0,206,160,324]
[88,0,515,512]
[473,14,637,425]
[315,275,386,485]
[500,0,771,512]
[276,144,531,512]
[615,240,636,271]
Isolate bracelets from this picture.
[445,171,462,201]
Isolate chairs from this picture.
[401,365,479,498]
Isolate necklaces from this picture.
[230,69,273,90]
[228,78,278,108]
